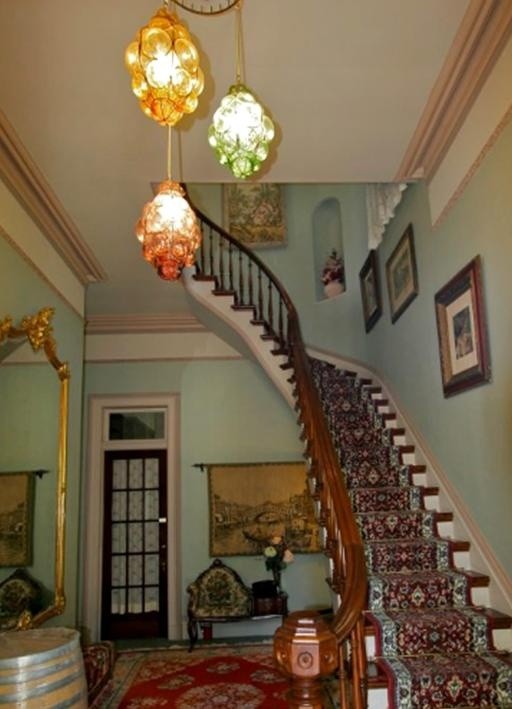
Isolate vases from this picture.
[322,278,344,298]
[271,571,283,595]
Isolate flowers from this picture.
[260,533,296,573]
[316,245,345,284]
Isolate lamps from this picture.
[117,0,281,284]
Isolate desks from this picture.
[251,590,289,627]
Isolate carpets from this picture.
[89,640,354,708]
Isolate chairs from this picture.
[181,554,257,654]
[1,565,49,628]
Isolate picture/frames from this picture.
[221,181,288,247]
[358,247,384,335]
[432,251,493,400]
[384,221,421,325]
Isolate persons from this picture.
[458,328,472,356]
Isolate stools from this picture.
[82,635,117,696]
